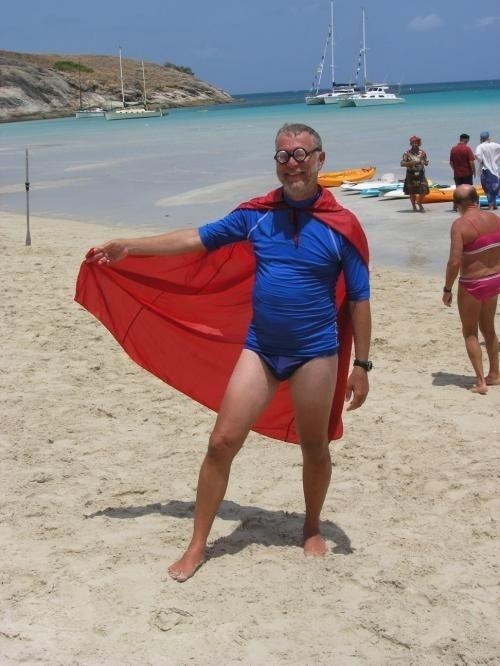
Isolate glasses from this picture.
[274,148,321,164]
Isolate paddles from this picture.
[26,148,31,245]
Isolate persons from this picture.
[474,131,500,210]
[449,133,477,212]
[442,183,500,394]
[399,135,430,213]
[83,123,371,582]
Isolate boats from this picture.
[316,166,500,207]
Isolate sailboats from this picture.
[303,1,406,107]
[72,46,168,119]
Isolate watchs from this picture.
[352,359,373,372]
[444,287,451,292]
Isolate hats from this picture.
[480,131,489,139]
[410,136,421,144]
